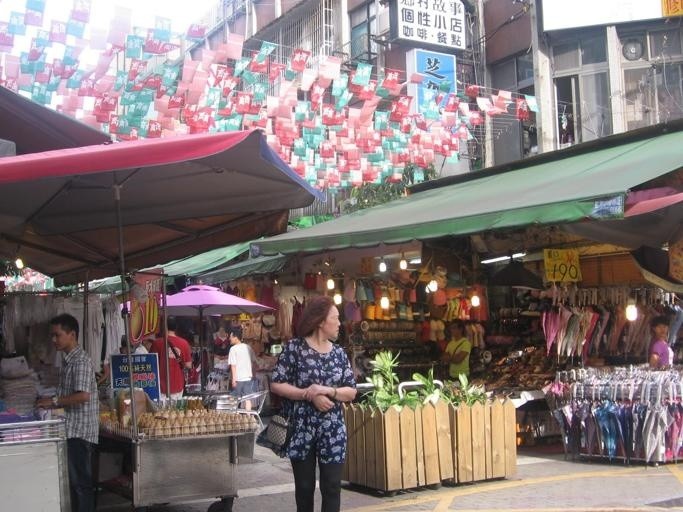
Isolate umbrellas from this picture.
[0,129,327,442]
[158,284,278,391]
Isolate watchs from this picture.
[51,396,58,407]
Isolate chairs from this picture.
[237,389,269,429]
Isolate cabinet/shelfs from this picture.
[89,412,256,512]
[0,419,73,512]
[332,274,430,382]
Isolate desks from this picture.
[186,391,230,410]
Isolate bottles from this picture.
[102,396,255,436]
[36,405,64,437]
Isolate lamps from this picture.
[470,287,480,308]
[327,250,438,310]
[625,293,638,321]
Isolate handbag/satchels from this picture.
[265,415,293,448]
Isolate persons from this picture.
[439,319,472,380]
[36,313,100,512]
[270,296,357,512]
[136,335,154,354]
[227,329,256,410]
[649,316,674,368]
[149,316,193,399]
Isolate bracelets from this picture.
[332,388,337,399]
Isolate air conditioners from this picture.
[375,6,391,38]
